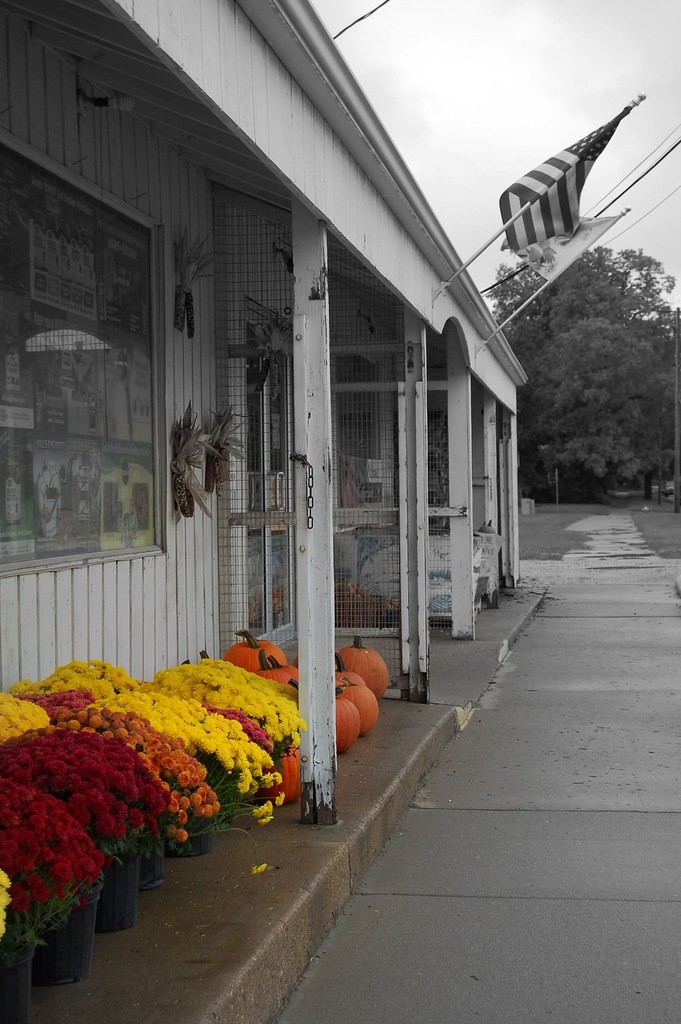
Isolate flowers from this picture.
[0,656,308,970]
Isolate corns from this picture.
[205,452,224,496]
[174,475,194,517]
[255,359,278,399]
[175,292,194,339]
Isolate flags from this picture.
[499,107,631,253]
[501,214,621,282]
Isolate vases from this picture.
[97,854,138,932]
[29,882,104,985]
[138,836,166,890]
[0,946,37,1024]
[166,807,212,856]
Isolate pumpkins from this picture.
[220,628,389,806]
